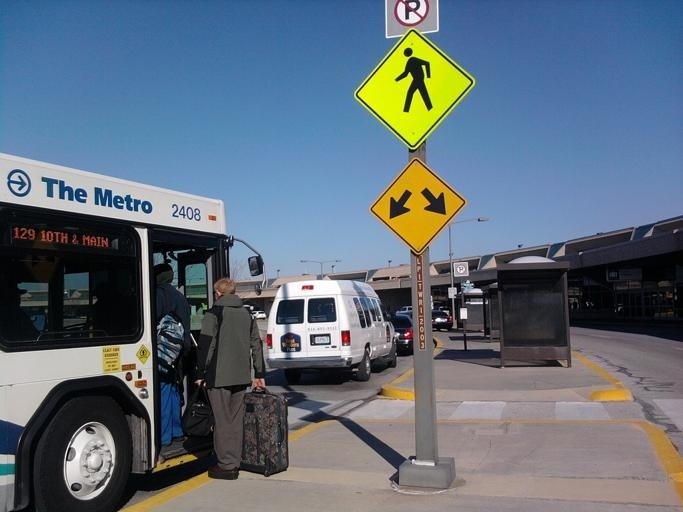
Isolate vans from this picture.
[265,277,397,381]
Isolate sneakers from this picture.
[160,436,184,455]
[208,464,239,480]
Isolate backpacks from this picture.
[156,310,185,383]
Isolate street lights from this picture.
[301,258,343,278]
[448,215,489,320]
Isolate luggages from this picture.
[240,385,289,477]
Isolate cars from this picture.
[251,310,266,320]
[393,306,412,356]
[431,310,453,331]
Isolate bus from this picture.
[1,154,265,512]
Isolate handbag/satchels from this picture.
[182,380,216,452]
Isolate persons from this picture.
[197,279,266,480]
[153,263,191,446]
